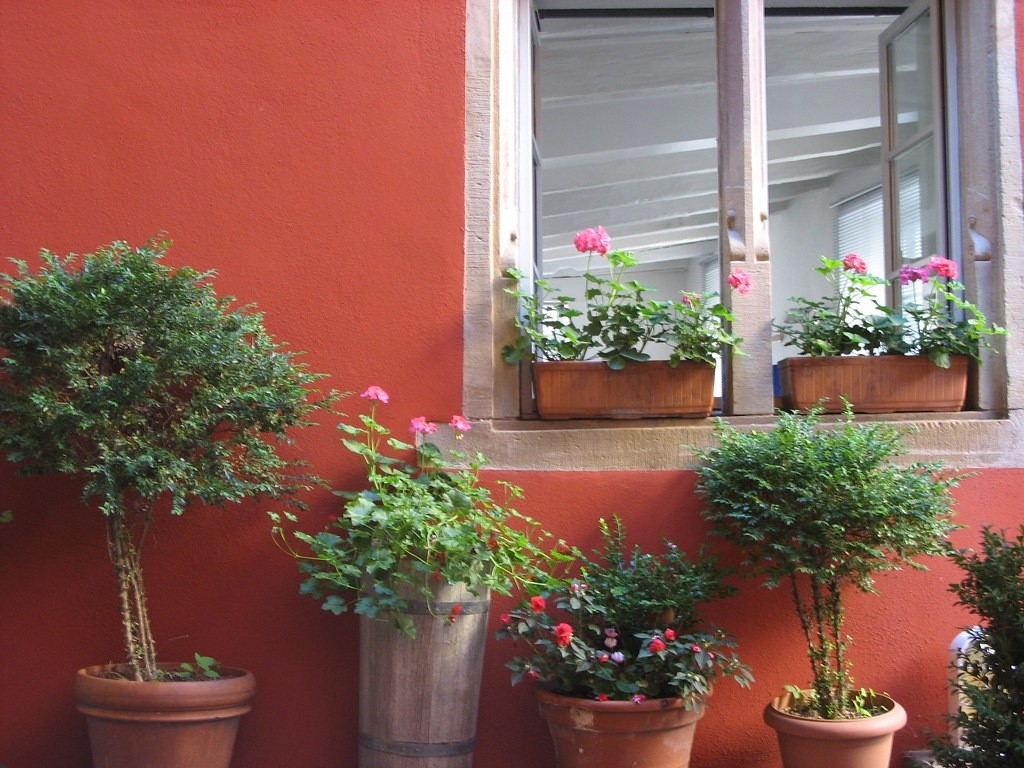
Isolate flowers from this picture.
[491,513,756,713]
[268,385,588,643]
[499,223,753,370]
[766,253,1008,370]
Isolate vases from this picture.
[354,551,492,768]
[775,353,970,414]
[535,674,713,768]
[529,358,717,420]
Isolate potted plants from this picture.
[0,229,355,768]
[684,390,982,768]
